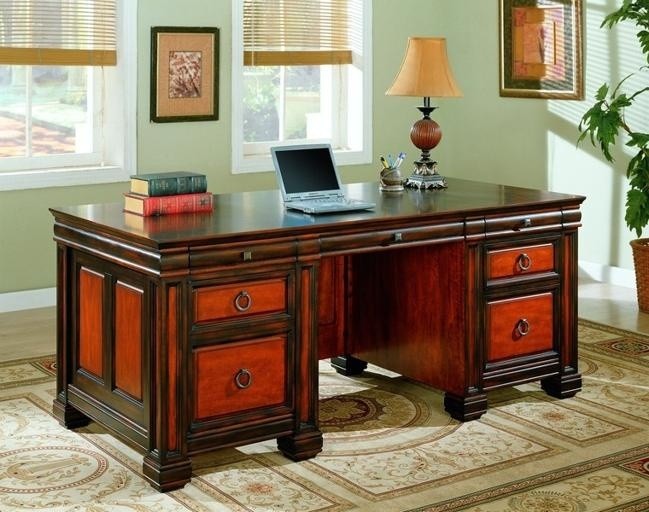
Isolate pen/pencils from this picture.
[380,152,407,169]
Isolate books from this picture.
[131,170,206,199]
[122,191,213,219]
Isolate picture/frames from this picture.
[150,24,220,124]
[498,1,584,101]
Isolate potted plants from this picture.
[575,1,648,317]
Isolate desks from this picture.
[49,176,586,494]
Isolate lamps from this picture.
[383,36,467,192]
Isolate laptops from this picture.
[269,143,378,215]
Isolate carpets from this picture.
[0,316,648,512]
